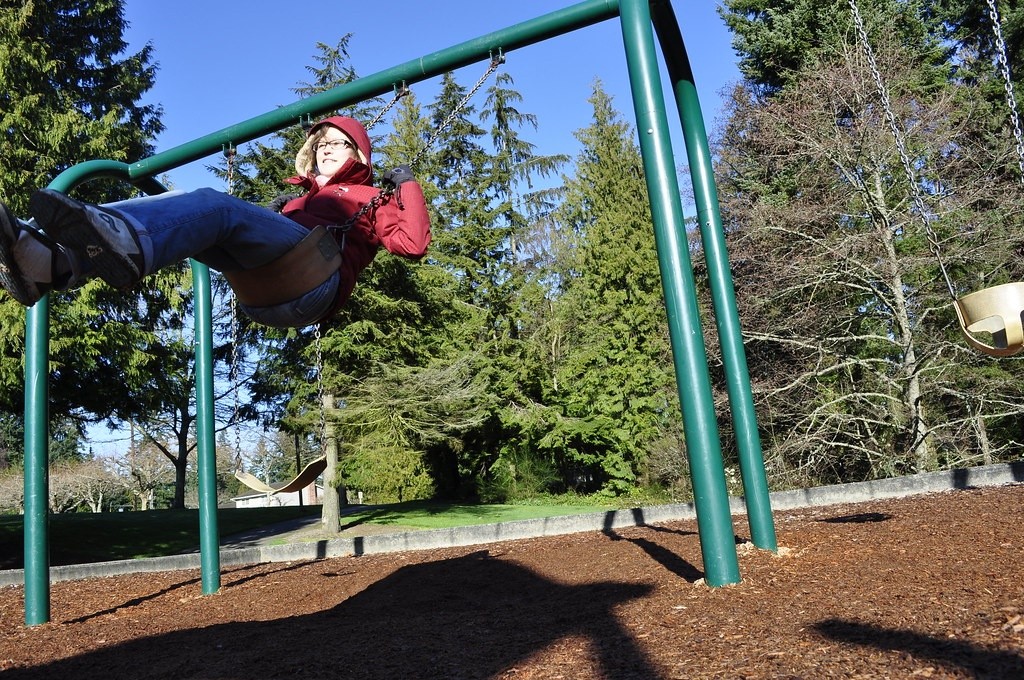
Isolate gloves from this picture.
[267,193,300,214]
[381,164,415,186]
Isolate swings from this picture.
[224,123,329,497]
[222,58,504,309]
[848,0,1024,357]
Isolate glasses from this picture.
[313,139,357,152]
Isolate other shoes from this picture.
[29,188,145,291]
[0,201,73,308]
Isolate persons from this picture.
[0,117,432,328]
